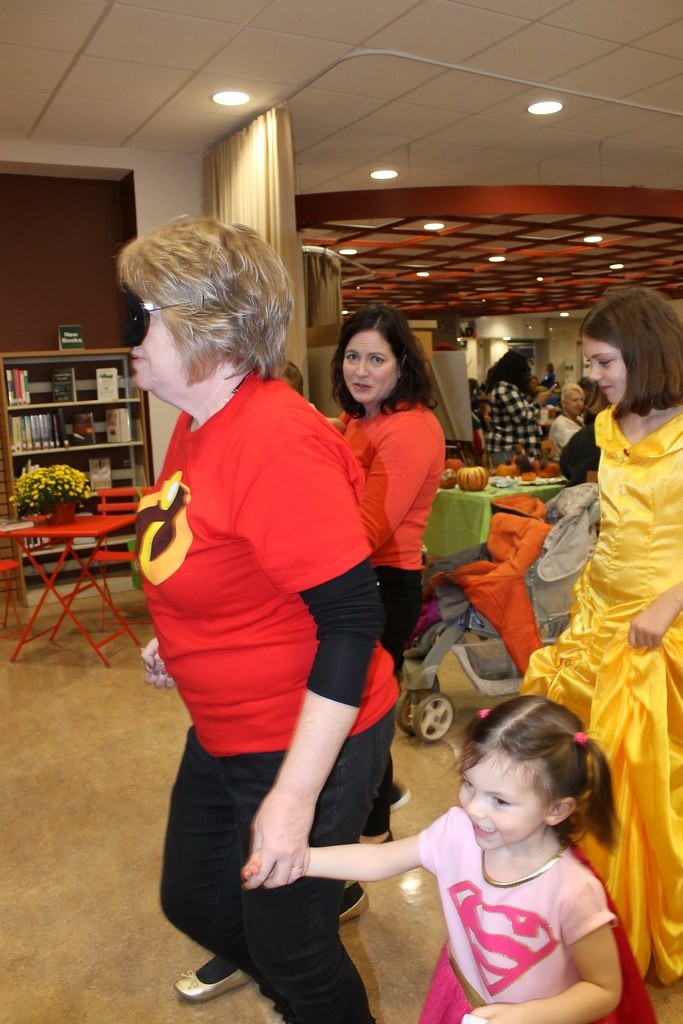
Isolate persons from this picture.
[317,301,447,922]
[528,361,556,406]
[241,693,622,1024]
[121,214,403,1024]
[519,301,683,996]
[277,354,348,436]
[545,376,602,489]
[468,348,553,470]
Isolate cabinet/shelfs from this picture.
[0,347,151,608]
[306,320,437,419]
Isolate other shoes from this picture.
[390,783,412,812]
[174,966,249,1003]
[337,884,371,923]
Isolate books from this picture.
[72,412,96,446]
[22,459,40,476]
[51,373,73,402]
[5,368,30,406]
[0,519,33,532]
[58,324,84,350]
[21,515,50,552]
[72,513,96,544]
[9,408,69,453]
[89,457,113,490]
[96,367,119,401]
[106,407,133,442]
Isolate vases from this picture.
[52,502,76,526]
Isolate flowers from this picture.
[9,464,91,521]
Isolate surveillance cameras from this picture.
[438,324,444,331]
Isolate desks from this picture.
[420,475,567,558]
[1,514,140,668]
[538,418,556,430]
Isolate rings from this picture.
[292,865,302,869]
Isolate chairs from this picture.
[94,486,154,631]
[0,558,25,638]
[457,439,598,483]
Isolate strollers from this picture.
[396,483,597,742]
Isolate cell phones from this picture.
[549,383,557,392]
